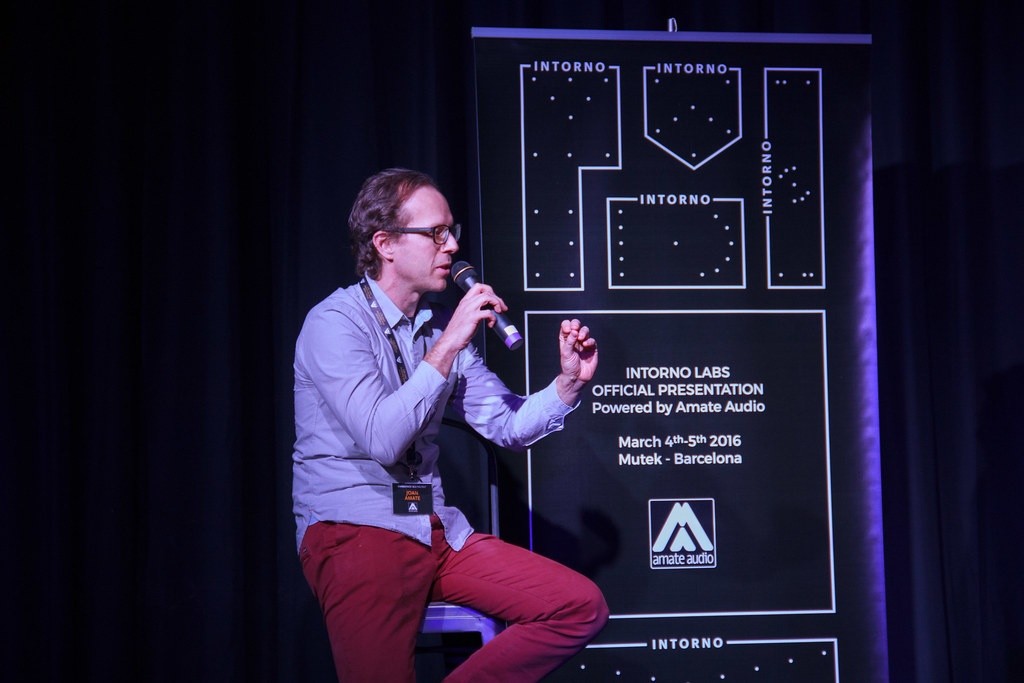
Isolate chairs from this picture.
[422,418,508,649]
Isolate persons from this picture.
[292,170,609,682]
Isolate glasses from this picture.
[380,223,461,244]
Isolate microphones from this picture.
[452,261,523,351]
